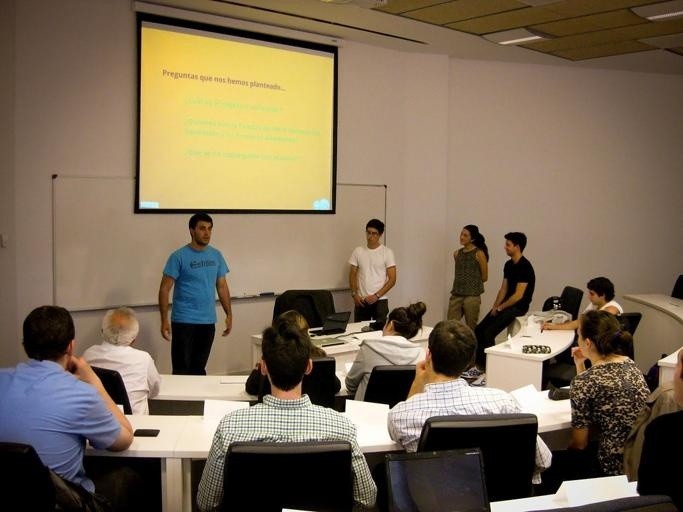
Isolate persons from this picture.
[348,219,396,330]
[568,311,652,479]
[345,302,426,402]
[198,319,377,512]
[387,320,552,484]
[447,225,489,331]
[1,305,161,511]
[540,277,623,389]
[637,349,682,511]
[461,232,535,385]
[159,214,232,375]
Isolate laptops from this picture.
[309,312,351,334]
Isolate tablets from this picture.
[311,338,344,346]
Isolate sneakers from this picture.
[460,365,487,387]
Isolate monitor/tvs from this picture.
[385,447,491,512]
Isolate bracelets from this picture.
[352,291,357,296]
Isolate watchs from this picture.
[375,293,380,299]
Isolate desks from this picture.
[491,481,641,510]
[482,310,577,394]
[152,371,353,403]
[81,382,575,509]
[622,292,682,376]
[249,318,438,395]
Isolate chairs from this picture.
[670,274,682,300]
[273,289,337,326]
[92,365,133,416]
[556,285,585,336]
[3,441,107,511]
[223,438,356,510]
[546,312,644,388]
[644,354,670,393]
[415,415,539,498]
[364,365,417,408]
